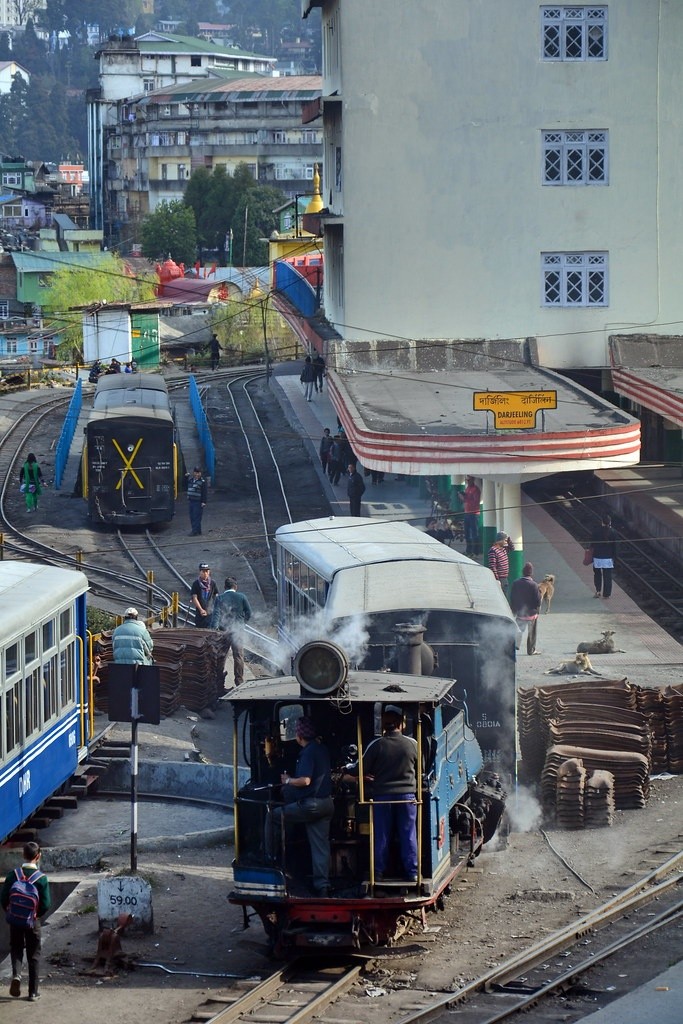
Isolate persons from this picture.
[4,841,50,1001]
[589,516,617,599]
[190,563,219,629]
[457,476,481,557]
[89,357,133,383]
[313,354,326,393]
[342,705,423,882]
[300,356,316,402]
[509,561,542,655]
[319,415,405,517]
[19,453,48,513]
[488,532,515,596]
[113,607,154,665]
[423,516,453,543]
[202,334,223,372]
[184,466,207,536]
[264,717,335,898]
[211,577,252,688]
[87,650,105,715]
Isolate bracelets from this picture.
[609,525,612,526]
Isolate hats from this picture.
[295,716,323,740]
[381,710,405,729]
[521,561,534,576]
[495,532,508,541]
[194,466,202,472]
[425,516,438,527]
[199,563,211,571]
[124,607,138,617]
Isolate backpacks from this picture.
[6,868,45,928]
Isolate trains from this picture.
[1,557,96,843]
[226,514,526,958]
[82,372,178,529]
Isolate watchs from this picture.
[285,777,291,784]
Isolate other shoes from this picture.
[603,593,611,597]
[187,530,202,536]
[26,505,38,512]
[10,974,22,997]
[29,992,41,1000]
[527,650,542,656]
[320,886,333,899]
[593,591,602,599]
[412,873,424,883]
[371,868,389,883]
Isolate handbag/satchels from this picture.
[583,548,597,566]
[20,483,37,493]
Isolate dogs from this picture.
[537,574,555,614]
[577,630,627,653]
[543,651,602,676]
